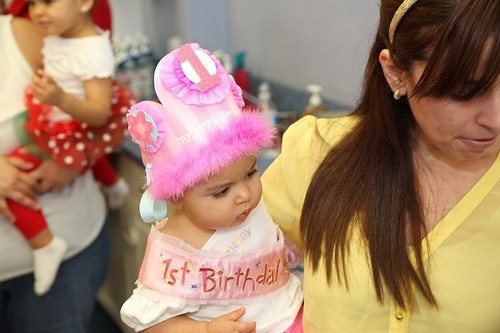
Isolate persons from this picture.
[261,0,500,333]
[120,44,305,333]
[0,14,109,333]
[0,0,130,297]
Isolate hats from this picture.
[125,42,278,198]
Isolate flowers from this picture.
[127,112,154,146]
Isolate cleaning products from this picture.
[302,85,326,117]
[258,83,280,158]
[116,32,158,100]
[234,50,252,103]
